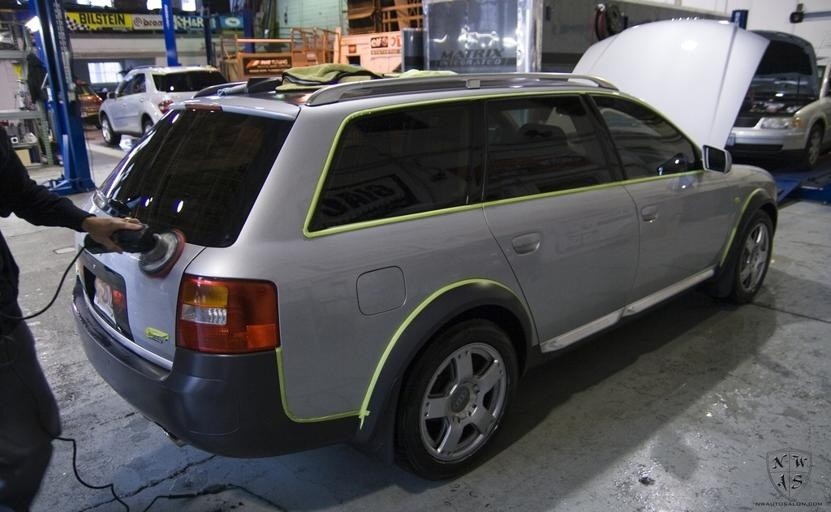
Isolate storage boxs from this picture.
[15,149,32,166]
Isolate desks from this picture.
[0,109,54,166]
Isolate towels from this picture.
[216,62,460,97]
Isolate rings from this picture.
[125,216,132,223]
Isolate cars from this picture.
[726,30,831,171]
[75,77,102,131]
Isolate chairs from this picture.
[512,121,589,189]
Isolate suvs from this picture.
[70,16,780,483]
[99,66,228,145]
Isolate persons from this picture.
[0,123,147,512]
